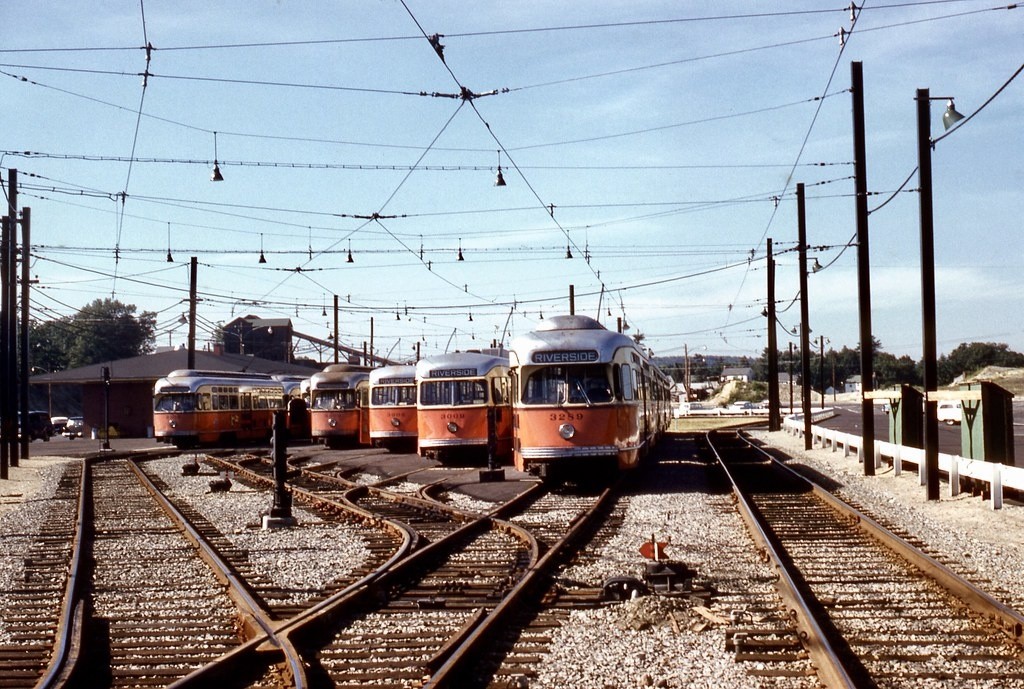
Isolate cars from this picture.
[18,412,82,442]
[937,399,963,425]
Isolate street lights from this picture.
[790,322,811,412]
[912,87,968,501]
[761,305,783,432]
[31,366,52,417]
[813,335,830,410]
[798,256,824,454]
[787,342,798,415]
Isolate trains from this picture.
[152,369,308,449]
[507,312,674,492]
[308,364,369,449]
[412,349,518,465]
[368,366,417,451]
